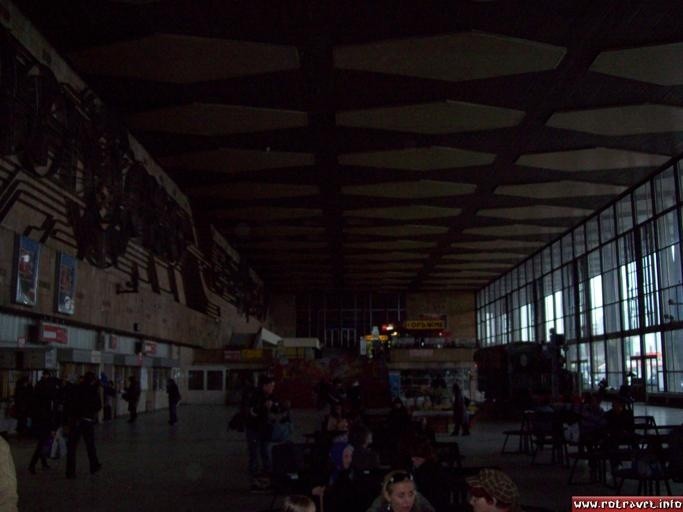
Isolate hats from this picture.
[466,468,521,503]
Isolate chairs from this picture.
[272,400,683,511]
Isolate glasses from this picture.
[386,472,414,491]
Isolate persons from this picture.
[0,321,683,512]
